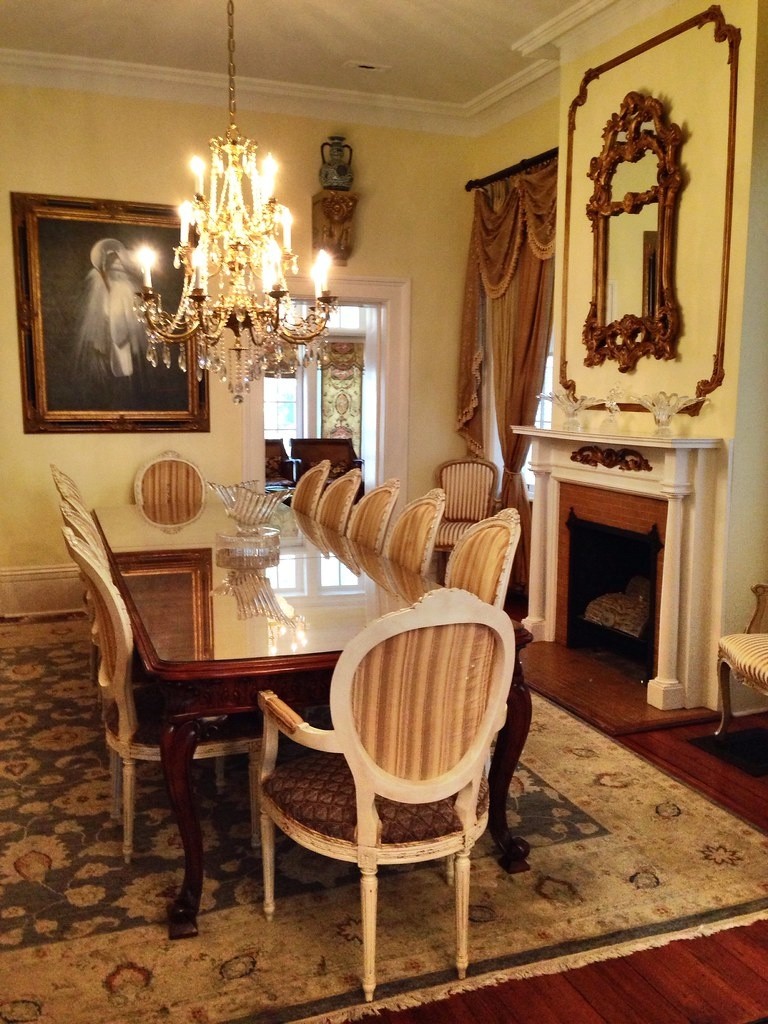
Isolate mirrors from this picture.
[581,90,684,373]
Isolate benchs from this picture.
[290,437,365,505]
[264,437,295,506]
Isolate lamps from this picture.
[132,1,341,406]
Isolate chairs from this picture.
[134,450,209,505]
[713,583,768,741]
[432,457,499,590]
[50,459,523,866]
[258,586,517,1003]
[138,495,206,535]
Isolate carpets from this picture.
[0,618,767,1024]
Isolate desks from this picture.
[90,498,532,941]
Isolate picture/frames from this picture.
[109,546,215,661]
[9,191,211,434]
